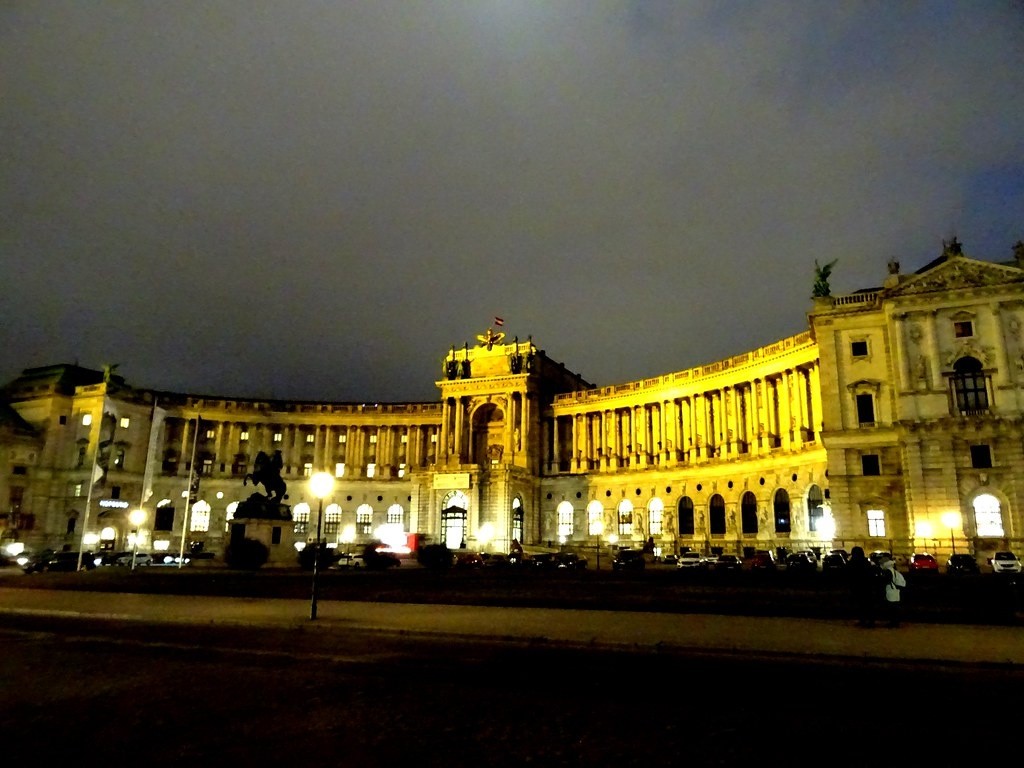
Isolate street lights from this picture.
[813,515,836,556]
[307,471,336,621]
[127,508,149,570]
[941,509,964,555]
[591,521,605,570]
[915,521,933,555]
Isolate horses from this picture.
[244,451,286,503]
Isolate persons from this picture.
[876,553,903,630]
[272,448,284,477]
[842,546,875,628]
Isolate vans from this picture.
[612,549,646,570]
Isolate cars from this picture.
[163,553,185,564]
[716,554,742,569]
[829,549,849,565]
[339,553,367,567]
[677,552,708,569]
[452,551,587,570]
[116,553,153,568]
[945,554,981,575]
[747,554,776,572]
[22,550,116,575]
[796,550,818,569]
[705,554,719,563]
[660,554,677,564]
[757,550,774,562]
[822,553,846,572]
[869,550,897,571]
[786,554,814,574]
[990,551,1022,575]
[908,552,939,572]
